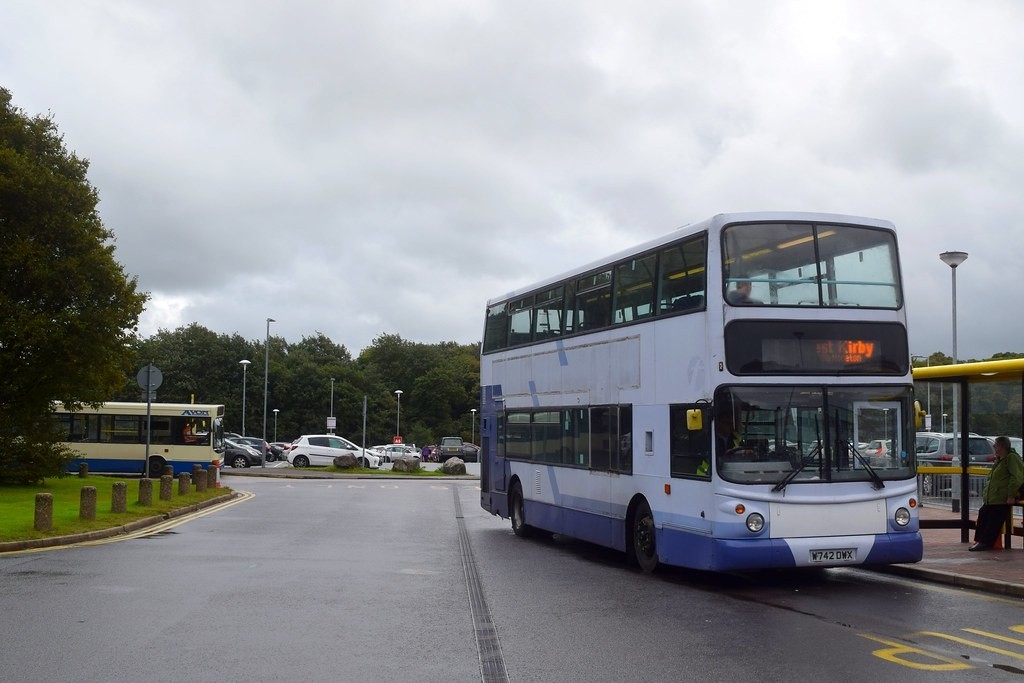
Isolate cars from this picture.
[769,432,1023,496]
[224,431,290,468]
[283,435,385,469]
[368,443,482,464]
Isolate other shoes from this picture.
[969,542,993,551]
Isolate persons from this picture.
[421,443,429,462]
[735,274,763,304]
[968,437,1024,551]
[183,424,199,442]
[702,411,752,463]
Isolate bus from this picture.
[479,210,923,575]
[32,401,225,478]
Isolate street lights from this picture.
[261,318,277,468]
[272,409,280,442]
[394,390,403,443]
[329,377,336,434]
[238,359,252,438]
[470,409,477,443]
[938,250,970,514]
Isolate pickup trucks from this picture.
[439,436,466,461]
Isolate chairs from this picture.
[649,302,674,316]
[546,323,599,338]
